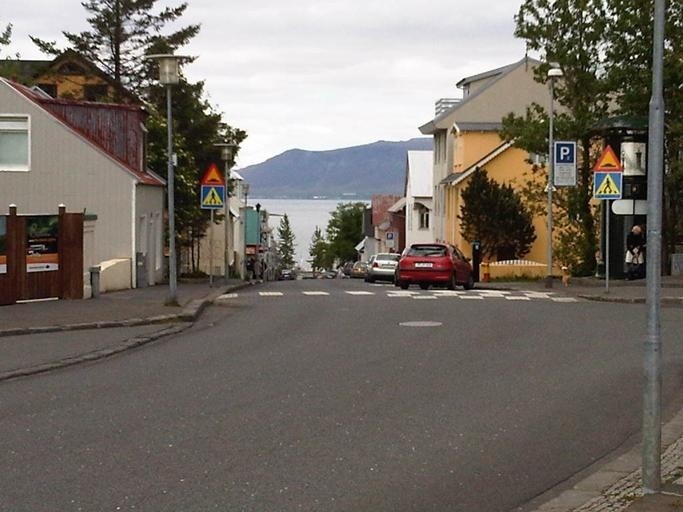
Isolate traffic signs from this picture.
[594,171,623,200]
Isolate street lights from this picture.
[146,54,190,307]
[548,69,563,288]
[214,141,252,283]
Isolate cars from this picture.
[278,269,297,280]
[398,242,474,290]
[302,253,402,287]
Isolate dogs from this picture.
[561,267,572,288]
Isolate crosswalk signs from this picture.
[201,186,225,210]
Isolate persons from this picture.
[246,257,266,280]
[625,225,643,280]
